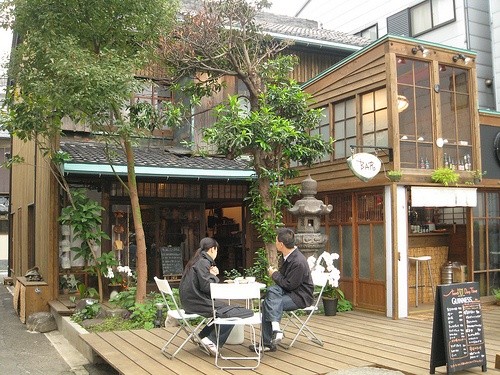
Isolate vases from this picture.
[108,283,120,295]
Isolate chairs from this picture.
[280,273,330,349]
[154,275,206,360]
[209,282,264,371]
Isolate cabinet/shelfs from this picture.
[182,220,243,271]
[14,277,48,323]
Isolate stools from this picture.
[408,256,436,307]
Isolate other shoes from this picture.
[191,334,203,348]
[270,329,285,345]
[201,336,220,356]
[249,341,276,353]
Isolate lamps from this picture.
[398,95,410,113]
[413,45,431,58]
[452,53,472,65]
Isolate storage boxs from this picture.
[453,264,466,284]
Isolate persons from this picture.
[249,229,314,353]
[179,238,235,358]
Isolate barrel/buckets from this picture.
[442,265,453,284]
[225,324,244,345]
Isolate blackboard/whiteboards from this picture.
[159,246,184,277]
[430,282,487,374]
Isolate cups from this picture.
[235,276,257,284]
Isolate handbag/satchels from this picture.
[216,304,254,319]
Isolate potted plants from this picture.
[322,284,338,316]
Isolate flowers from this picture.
[103,265,132,283]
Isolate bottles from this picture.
[444,155,472,171]
[420,159,425,168]
[411,223,428,233]
[425,159,430,169]
